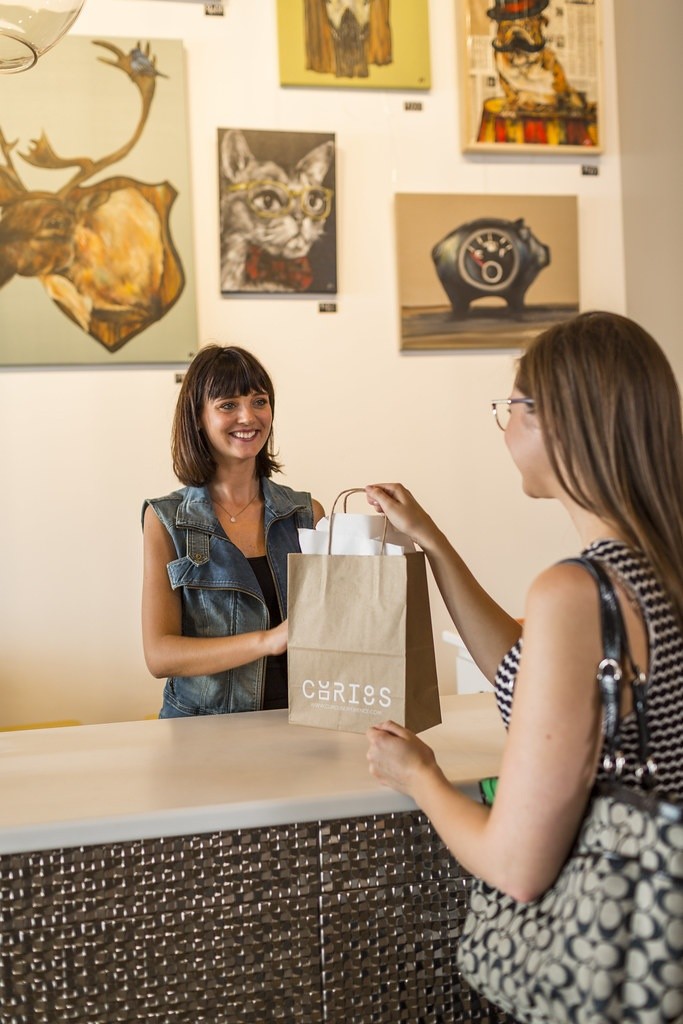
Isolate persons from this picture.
[368,312,683,905]
[141,346,325,720]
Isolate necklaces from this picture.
[212,492,260,523]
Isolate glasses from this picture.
[492,399,536,430]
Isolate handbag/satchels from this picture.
[287,487,442,737]
[457,557,683,1024]
